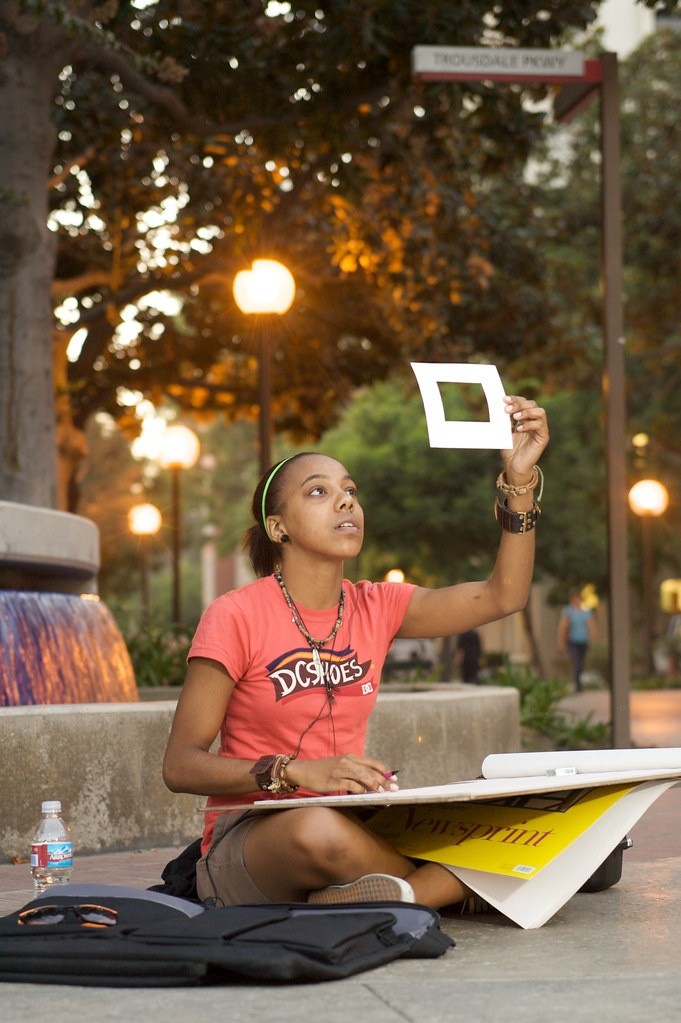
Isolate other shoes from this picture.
[307,872,416,905]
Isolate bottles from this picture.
[29,800,73,898]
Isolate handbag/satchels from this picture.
[0,884,457,988]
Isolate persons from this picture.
[663,593,681,674]
[456,631,481,683]
[387,638,434,678]
[163,396,549,911]
[559,591,596,690]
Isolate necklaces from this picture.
[276,572,345,645]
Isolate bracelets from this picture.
[280,753,299,792]
[250,755,273,791]
[495,497,539,533]
[496,465,544,500]
[269,754,288,794]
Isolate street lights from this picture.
[155,425,203,630]
[627,480,670,677]
[129,503,162,630]
[233,258,295,480]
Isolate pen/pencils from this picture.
[344,769,402,797]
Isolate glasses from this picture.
[17,903,118,928]
